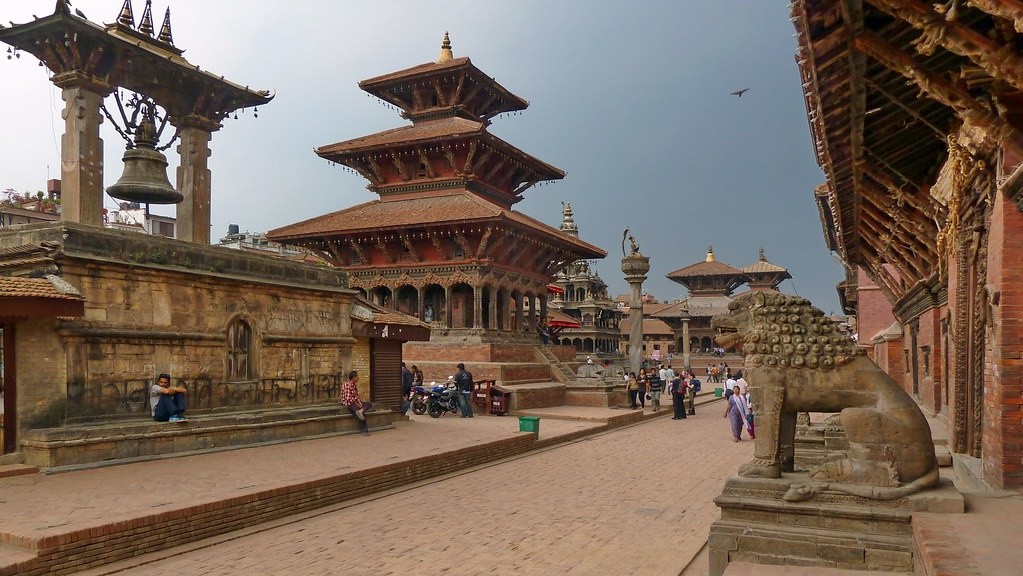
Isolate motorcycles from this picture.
[408,375,459,418]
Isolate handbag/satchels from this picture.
[725,389,734,397]
[646,393,651,400]
[691,380,701,392]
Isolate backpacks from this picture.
[674,378,687,394]
[706,368,708,373]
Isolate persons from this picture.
[595,345,627,358]
[666,352,673,364]
[615,301,630,309]
[511,314,559,346]
[624,363,697,419]
[706,362,747,400]
[491,390,503,417]
[341,371,372,436]
[585,356,594,364]
[705,346,725,359]
[630,237,640,254]
[454,363,475,417]
[401,362,423,416]
[724,386,755,442]
[150,374,189,423]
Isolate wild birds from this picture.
[731,88,749,97]
[75,8,87,20]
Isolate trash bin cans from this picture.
[473,379,497,411]
[715,388,722,397]
[518,416,541,441]
[490,386,511,417]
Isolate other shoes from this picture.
[363,431,369,435]
[734,438,738,442]
[632,407,634,409]
[459,415,466,418]
[640,406,642,408]
[642,408,644,410]
[672,415,686,420]
[635,407,637,409]
[468,416,473,418]
[356,410,365,420]
[738,436,741,440]
[169,415,187,423]
